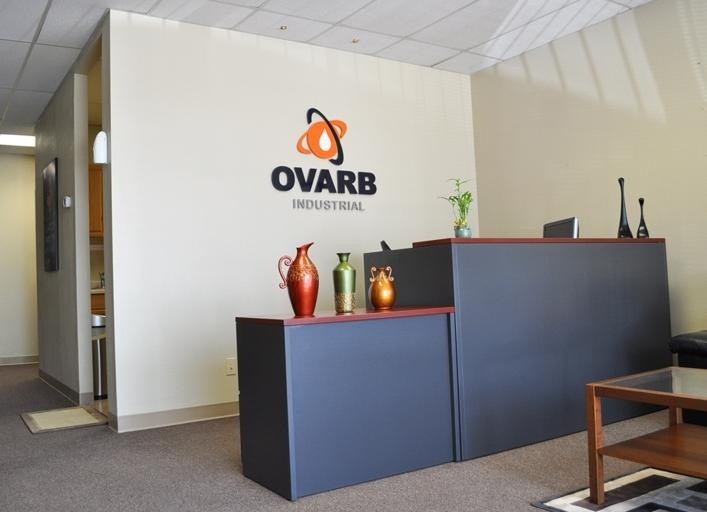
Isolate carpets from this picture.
[18,405,108,435]
[530,466,707,512]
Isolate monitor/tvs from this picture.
[542,217,579,238]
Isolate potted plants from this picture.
[438,177,474,238]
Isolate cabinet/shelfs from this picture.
[90,165,103,238]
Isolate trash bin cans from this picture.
[91,313,108,401]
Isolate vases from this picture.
[278,241,397,318]
